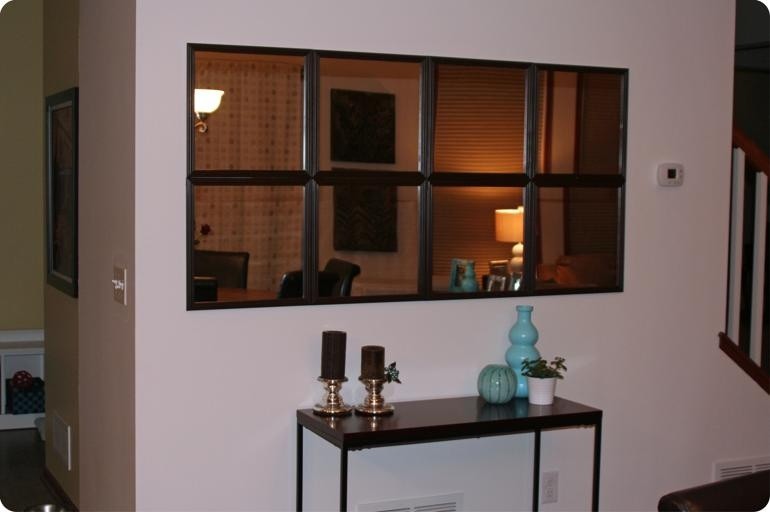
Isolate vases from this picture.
[462,263,478,292]
[506,306,541,399]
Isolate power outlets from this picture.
[542,472,557,502]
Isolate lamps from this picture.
[194,89,225,132]
[496,206,524,276]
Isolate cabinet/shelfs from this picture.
[719,106,770,397]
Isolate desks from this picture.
[197,287,281,307]
[296,396,602,511]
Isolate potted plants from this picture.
[521,357,567,405]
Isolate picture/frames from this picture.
[450,258,475,293]
[486,270,510,292]
[46,86,80,299]
[488,260,509,276]
[509,272,522,291]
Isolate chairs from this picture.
[322,258,360,299]
[193,250,249,290]
[277,271,321,299]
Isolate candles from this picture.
[361,345,384,379]
[321,330,346,379]
